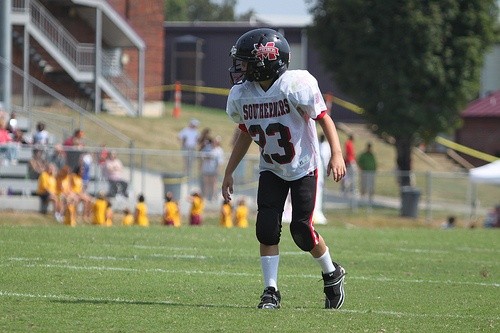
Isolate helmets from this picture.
[229,28,291,82]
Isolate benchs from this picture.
[0,113,132,211]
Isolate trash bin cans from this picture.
[162,174,183,202]
[401,187,420,217]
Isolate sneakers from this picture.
[322,261,346,309]
[257,287,281,310]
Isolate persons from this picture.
[178,118,202,178]
[121,207,135,226]
[222,29,347,310]
[341,134,357,196]
[358,143,379,213]
[220,199,235,228]
[187,192,205,225]
[233,198,250,229]
[1,111,128,220]
[199,136,225,203]
[91,192,115,226]
[135,195,150,227]
[318,133,333,188]
[162,193,183,226]
